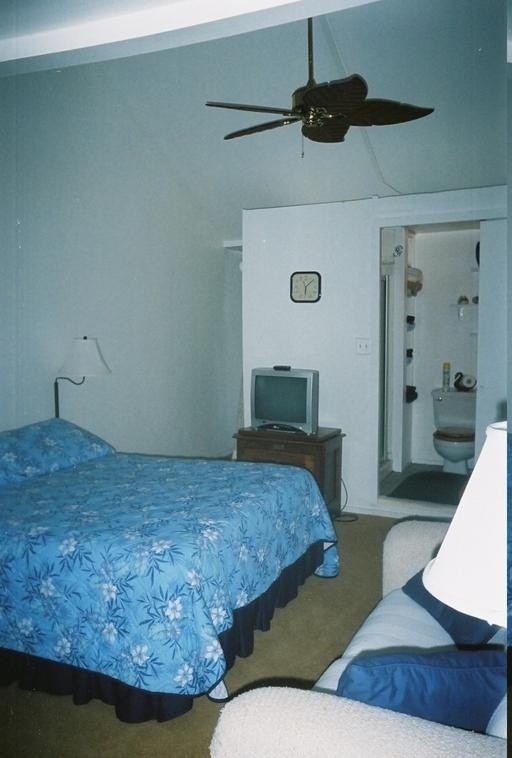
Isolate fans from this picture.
[204,15,439,143]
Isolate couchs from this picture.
[208,517,506,758]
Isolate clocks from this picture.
[291,272,322,302]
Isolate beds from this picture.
[0,419,340,723]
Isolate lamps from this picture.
[53,335,111,419]
[423,417,506,632]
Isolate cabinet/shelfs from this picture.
[232,425,347,521]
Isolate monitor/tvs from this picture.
[251,368,319,435]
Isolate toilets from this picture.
[431,386,476,475]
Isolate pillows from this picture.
[335,647,507,732]
[1,417,116,485]
[402,538,502,650]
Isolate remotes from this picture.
[274,365,289,370]
